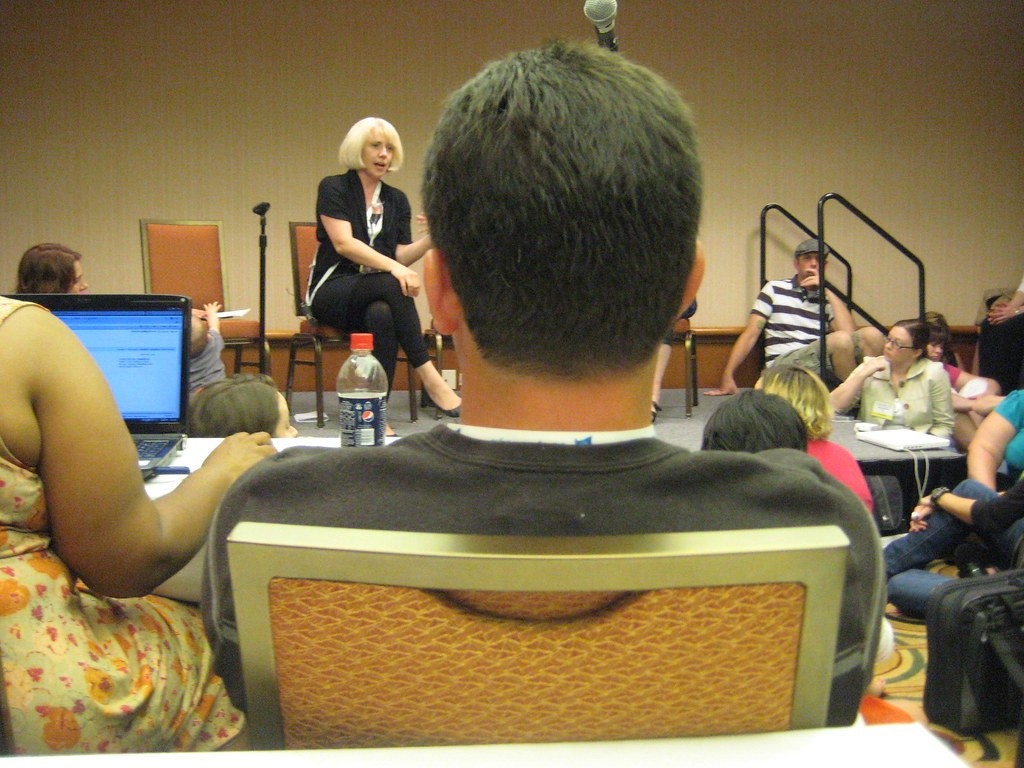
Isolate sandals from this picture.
[651,401,662,422]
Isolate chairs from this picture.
[139,217,272,375]
[224,521,851,750]
[283,221,419,427]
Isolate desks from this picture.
[124,436,406,605]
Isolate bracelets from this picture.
[1016,307,1021,315]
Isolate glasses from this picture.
[883,335,915,350]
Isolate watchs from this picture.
[930,487,950,508]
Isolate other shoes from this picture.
[421,383,462,417]
[383,426,398,438]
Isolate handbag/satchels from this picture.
[923,567,1024,735]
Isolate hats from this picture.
[795,236,829,256]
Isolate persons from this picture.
[754,364,872,508]
[979,277,1024,395]
[15,243,88,293]
[0,294,278,758]
[830,312,1007,451]
[701,388,808,453]
[966,388,1024,495]
[883,472,1024,620]
[189,374,297,438]
[199,40,886,727]
[190,301,226,392]
[650,298,697,421]
[305,116,462,418]
[704,240,885,395]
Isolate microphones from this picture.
[584,0,619,53]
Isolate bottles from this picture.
[335,332,389,451]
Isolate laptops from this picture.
[0,293,192,483]
[855,429,951,452]
